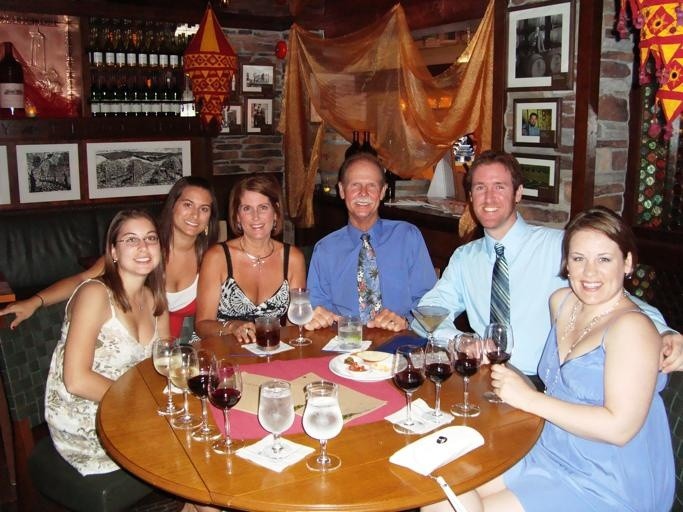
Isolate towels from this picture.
[388,426,485,476]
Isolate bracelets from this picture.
[216,318,231,336]
[36,292,46,307]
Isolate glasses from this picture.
[115,235,160,247]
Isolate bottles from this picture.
[0,42,25,119]
[345,129,396,203]
[87,23,195,117]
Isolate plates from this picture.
[327,351,408,381]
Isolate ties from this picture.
[356,234,383,325]
[489,243,510,352]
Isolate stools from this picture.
[28,434,154,511]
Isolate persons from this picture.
[528,26,546,55]
[253,102,266,128]
[523,113,542,135]
[0,175,222,347]
[42,210,223,511]
[421,206,675,512]
[246,72,266,85]
[195,177,307,344]
[411,150,682,374]
[304,151,440,331]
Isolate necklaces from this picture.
[239,238,276,270]
[542,289,630,395]
[124,288,145,312]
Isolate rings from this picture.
[390,319,396,326]
[246,325,253,333]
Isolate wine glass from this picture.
[302,381,342,473]
[185,349,222,442]
[452,332,483,419]
[150,337,187,417]
[420,341,452,424]
[258,381,295,458]
[286,288,313,347]
[205,358,245,456]
[483,323,513,404]
[166,347,202,431]
[392,346,427,435]
[410,292,453,350]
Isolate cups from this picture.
[253,315,281,349]
[336,315,362,348]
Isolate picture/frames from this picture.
[83,135,199,207]
[509,152,559,201]
[500,1,577,92]
[512,98,561,145]
[0,139,15,208]
[219,104,244,135]
[244,95,274,135]
[240,62,275,94]
[14,139,84,208]
